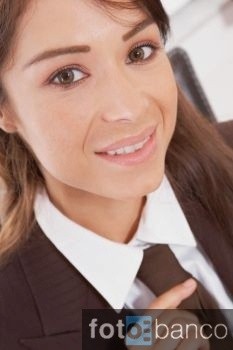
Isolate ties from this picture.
[135,243,232,350]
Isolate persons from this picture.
[0,0,233,350]
